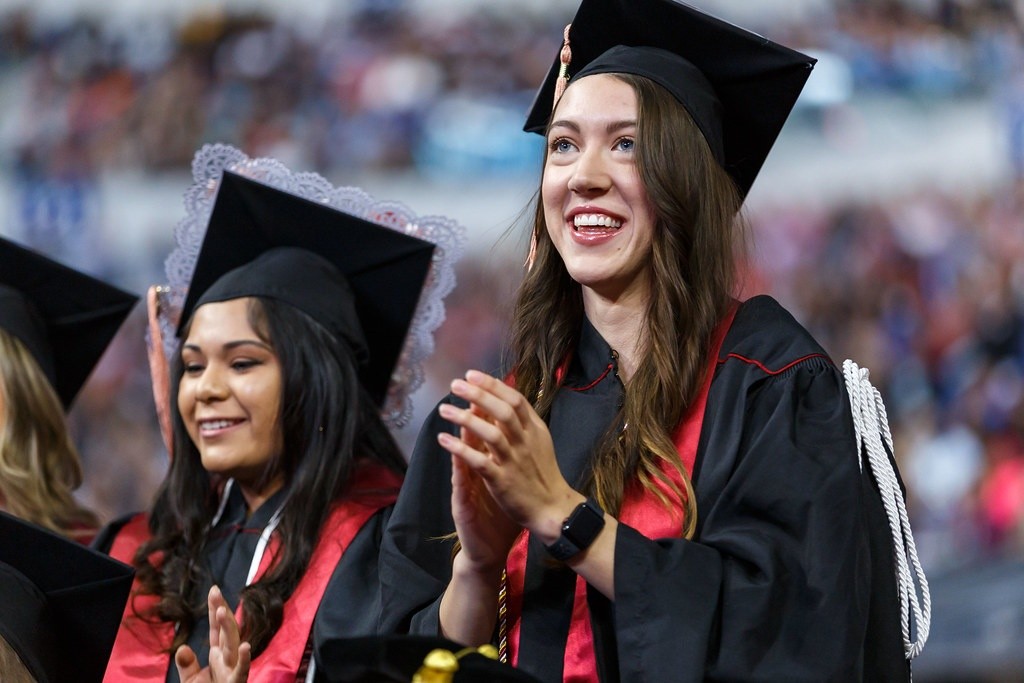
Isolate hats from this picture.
[144,141,467,458]
[0,236,140,416]
[523,0,818,217]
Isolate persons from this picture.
[0,511,137,683]
[0,234,142,549]
[375,1,932,682]
[89,143,468,683]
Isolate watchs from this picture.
[543,497,605,562]
